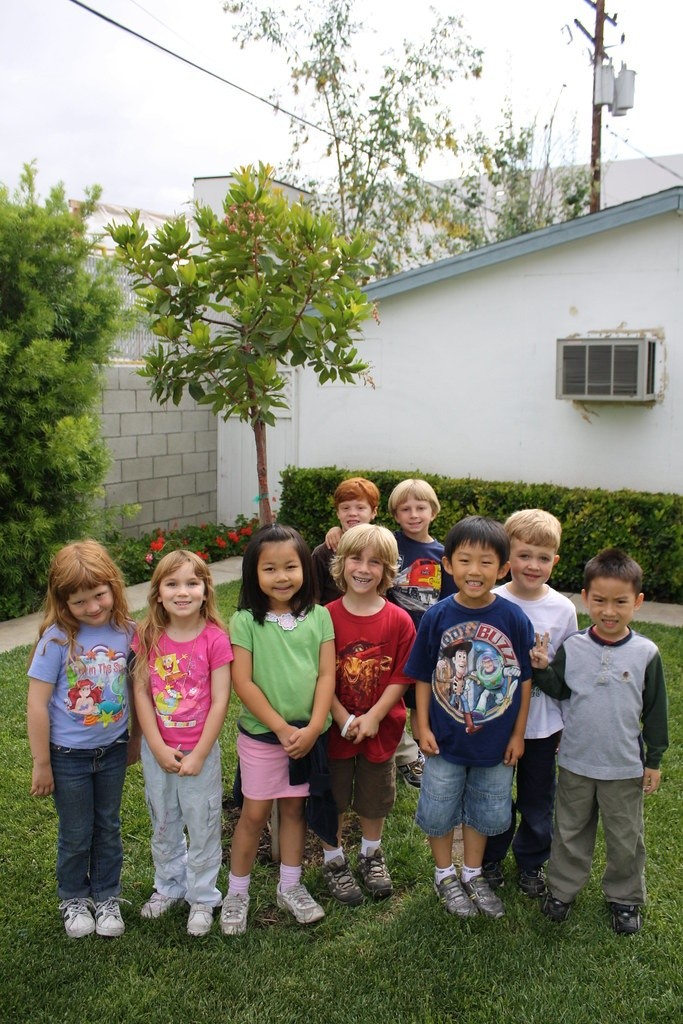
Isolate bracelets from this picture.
[340,715,355,737]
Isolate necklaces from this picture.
[164,618,200,699]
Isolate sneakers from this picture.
[59,898,97,938]
[433,874,479,918]
[541,892,575,923]
[91,896,132,936]
[322,855,365,906]
[276,882,325,924]
[518,869,547,896]
[397,750,426,789]
[220,893,250,935]
[187,903,213,936]
[483,862,504,889]
[609,901,641,935]
[460,873,506,918]
[356,847,393,897]
[140,892,178,919]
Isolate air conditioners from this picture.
[555,336,656,402]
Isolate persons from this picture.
[529,548,667,933]
[219,523,335,937]
[483,508,580,898]
[130,551,234,936]
[403,515,536,917]
[28,544,140,935]
[324,523,418,906]
[310,477,425,792]
[325,480,460,746]
